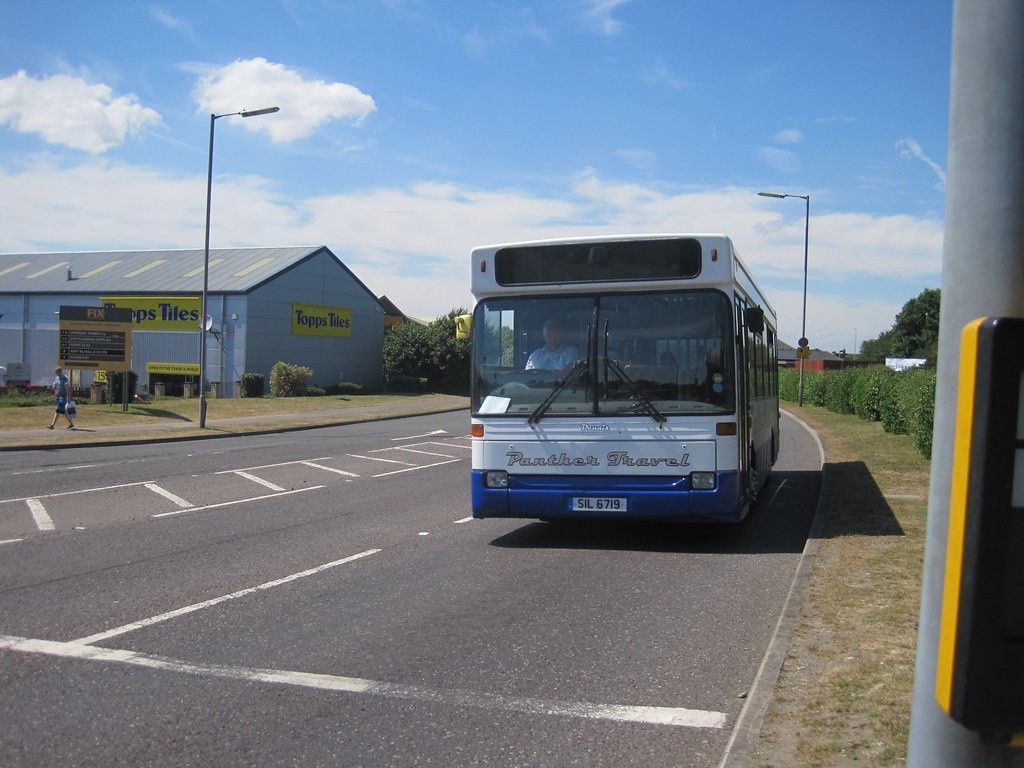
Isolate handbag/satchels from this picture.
[65,401,77,420]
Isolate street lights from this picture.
[757,191,810,407]
[200,107,280,427]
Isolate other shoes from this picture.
[46,424,54,429]
[66,424,74,428]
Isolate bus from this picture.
[453,233,777,528]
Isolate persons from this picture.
[46,367,74,429]
[524,318,579,370]
